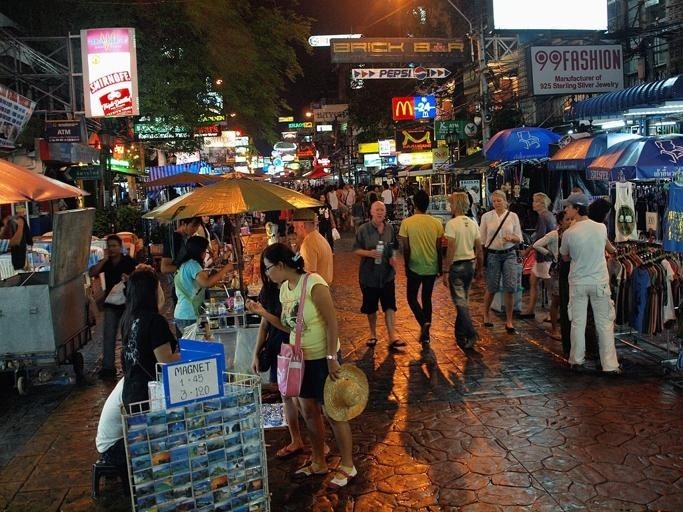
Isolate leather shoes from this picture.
[517,313,536,319]
[483,318,494,326]
[292,464,329,481]
[504,323,516,331]
[545,329,562,340]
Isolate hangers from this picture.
[611,240,676,272]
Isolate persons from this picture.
[354,202,407,348]
[511,185,523,219]
[365,185,378,217]
[88,235,138,378]
[469,185,480,218]
[250,244,330,465]
[277,178,327,194]
[373,187,382,202]
[326,186,342,228]
[503,181,513,205]
[521,193,555,322]
[389,183,398,200]
[355,184,364,203]
[95,376,129,466]
[251,208,289,243]
[398,191,444,344]
[210,212,225,245]
[172,237,232,336]
[120,269,180,413]
[121,192,131,205]
[381,184,394,217]
[562,194,623,373]
[345,184,355,226]
[480,191,522,332]
[534,211,577,340]
[194,215,212,270]
[292,209,333,287]
[563,198,613,358]
[442,193,482,346]
[170,188,179,201]
[0,209,33,269]
[171,217,201,259]
[245,244,358,489]
[315,195,336,256]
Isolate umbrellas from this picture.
[585,132,683,183]
[144,171,224,184]
[547,132,643,170]
[483,127,561,160]
[0,157,92,217]
[142,179,325,293]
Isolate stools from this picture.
[92,459,130,501]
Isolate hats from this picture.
[559,192,590,207]
[288,209,316,221]
[323,363,369,422]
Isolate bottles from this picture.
[209,298,219,329]
[218,301,227,329]
[234,291,245,330]
[374,241,384,264]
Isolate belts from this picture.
[452,257,477,264]
[488,244,516,254]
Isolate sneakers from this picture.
[464,334,479,349]
[454,336,466,346]
[424,337,431,342]
[419,323,431,344]
[326,465,358,488]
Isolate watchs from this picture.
[444,269,449,273]
[325,353,337,360]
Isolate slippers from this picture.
[389,339,407,348]
[366,338,378,346]
[275,445,306,461]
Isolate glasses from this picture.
[263,262,281,272]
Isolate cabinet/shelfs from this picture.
[205,231,269,324]
